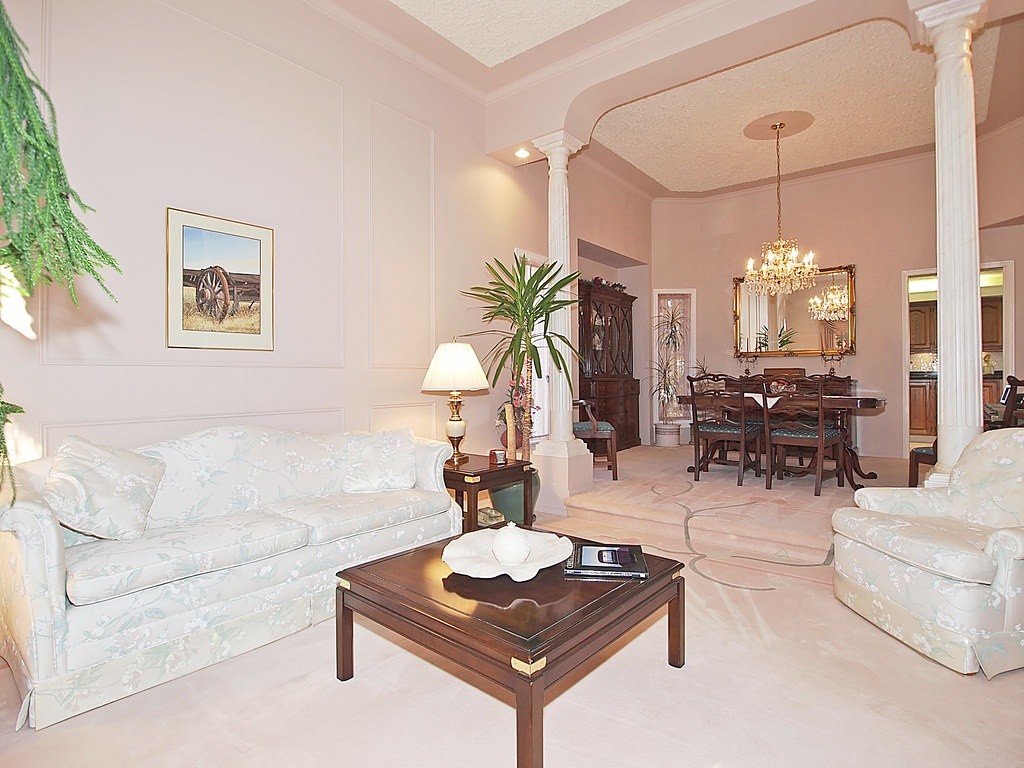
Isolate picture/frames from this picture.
[166,208,275,351]
[999,385,1011,404]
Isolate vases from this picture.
[501,427,523,450]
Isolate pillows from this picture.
[331,428,417,493]
[39,435,167,543]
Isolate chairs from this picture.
[687,369,850,496]
[909,376,1024,488]
[572,400,617,480]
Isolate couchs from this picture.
[0,425,462,730]
[830,428,1024,679]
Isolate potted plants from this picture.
[641,300,711,447]
[460,248,585,524]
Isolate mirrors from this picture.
[731,264,857,359]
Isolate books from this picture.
[563,542,649,580]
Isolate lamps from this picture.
[743,110,820,298]
[808,274,848,322]
[421,335,490,464]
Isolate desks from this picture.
[677,394,886,491]
[443,453,534,530]
[335,519,685,768]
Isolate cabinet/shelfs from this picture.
[909,296,1003,441]
[577,278,641,457]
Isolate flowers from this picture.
[493,368,541,433]
